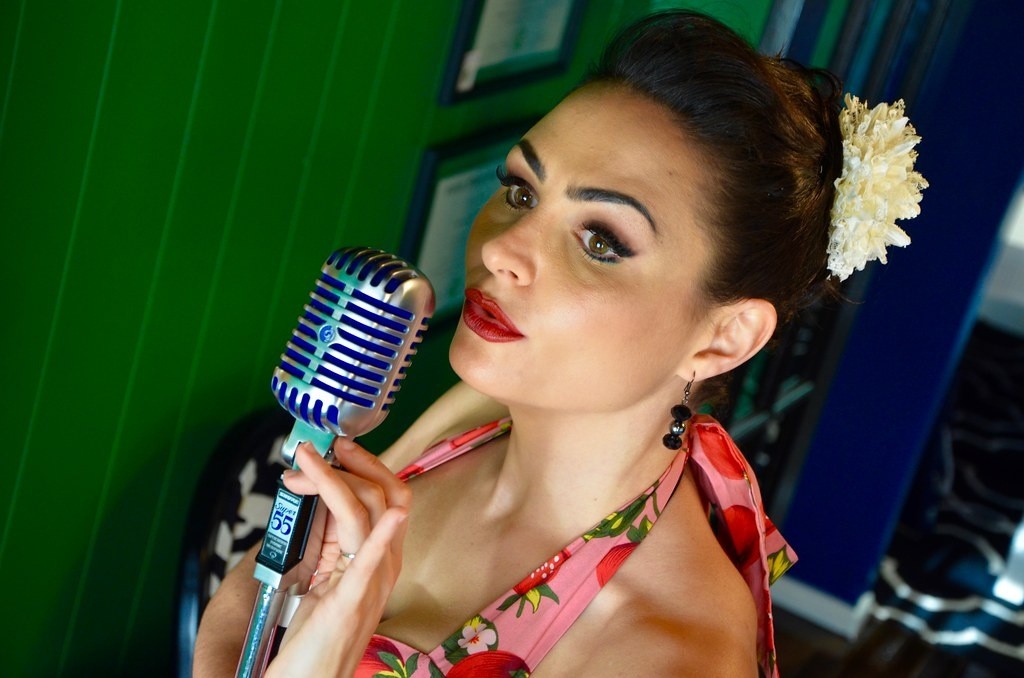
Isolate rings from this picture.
[340,549,356,562]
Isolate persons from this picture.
[191,7,931,678]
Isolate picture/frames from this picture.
[397,113,543,347]
[438,0,590,109]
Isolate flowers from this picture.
[822,90,929,281]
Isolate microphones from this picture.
[253,245,437,590]
